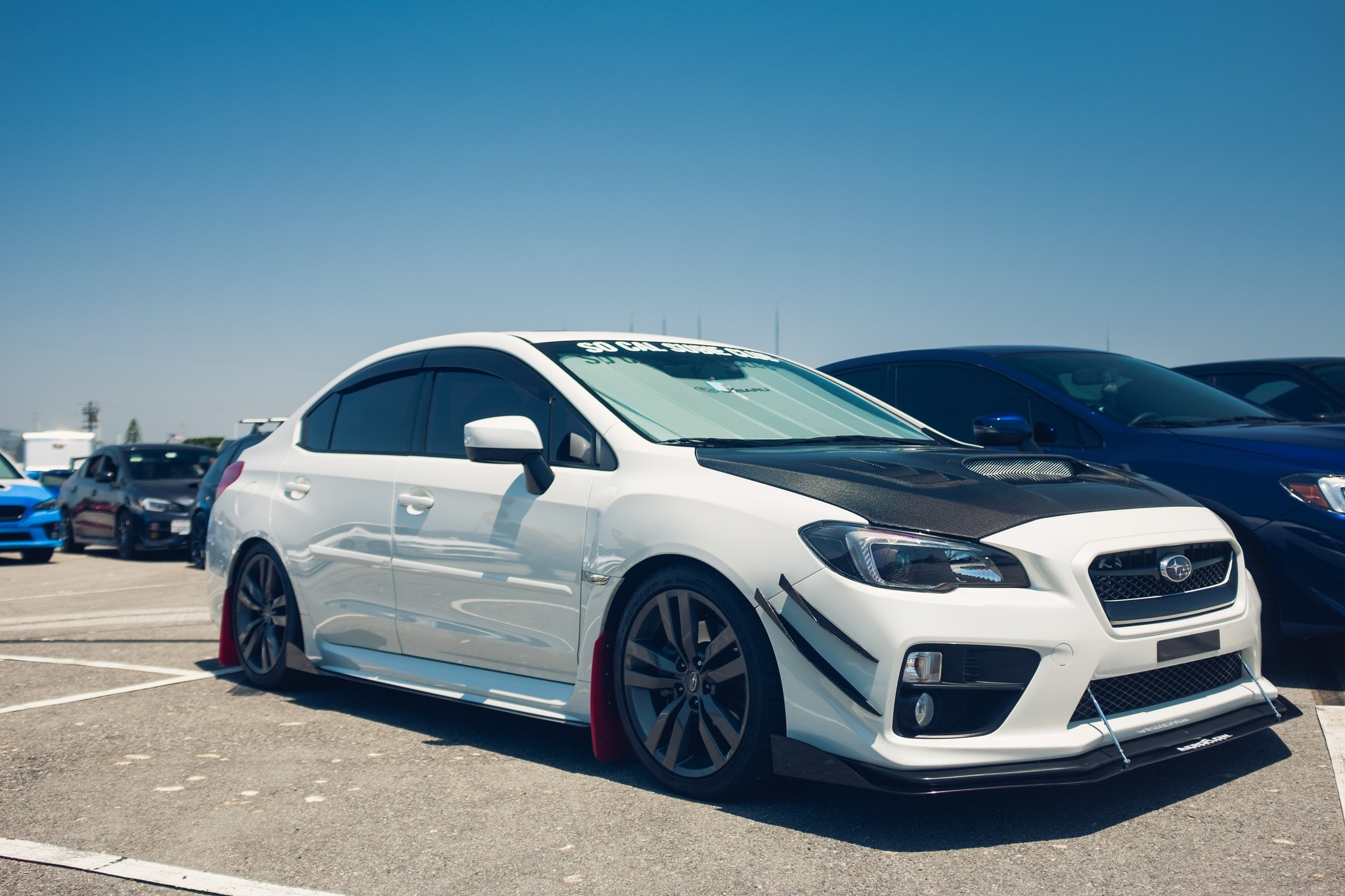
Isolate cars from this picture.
[24,470,75,498]
[1170,357,1345,419]
[203,331,1304,806]
[0,451,66,563]
[58,443,217,559]
[815,347,1345,684]
[190,418,288,566]
